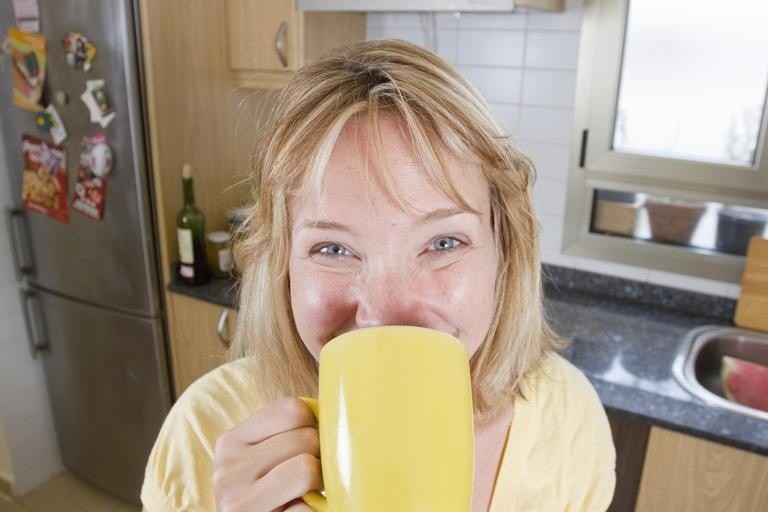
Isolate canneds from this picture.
[225,207,252,243]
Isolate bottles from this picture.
[176,164,208,286]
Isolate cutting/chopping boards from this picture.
[732,236,767,333]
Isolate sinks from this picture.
[671,323,767,418]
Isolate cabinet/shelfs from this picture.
[221,2,368,92]
[610,415,766,512]
[172,289,240,407]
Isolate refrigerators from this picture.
[0,1,175,505]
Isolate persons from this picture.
[138,37,620,512]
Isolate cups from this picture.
[280,326,476,512]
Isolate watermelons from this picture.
[721,354,768,414]
